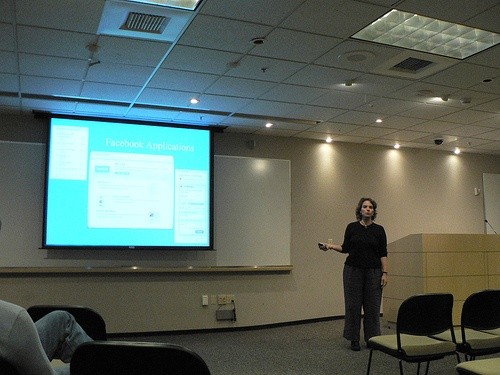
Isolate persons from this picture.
[0,299,94,375]
[319,198,388,351]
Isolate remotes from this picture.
[318,243,327,250]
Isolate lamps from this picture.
[433,137,443,145]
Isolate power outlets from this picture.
[217,294,228,305]
[226,294,235,303]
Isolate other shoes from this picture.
[351,341,361,351]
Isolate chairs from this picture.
[367,292,461,375]
[456,357,500,375]
[69,341,211,374]
[426,288,500,375]
[25,303,106,341]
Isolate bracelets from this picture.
[383,272,388,273]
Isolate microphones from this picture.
[485,220,496,234]
